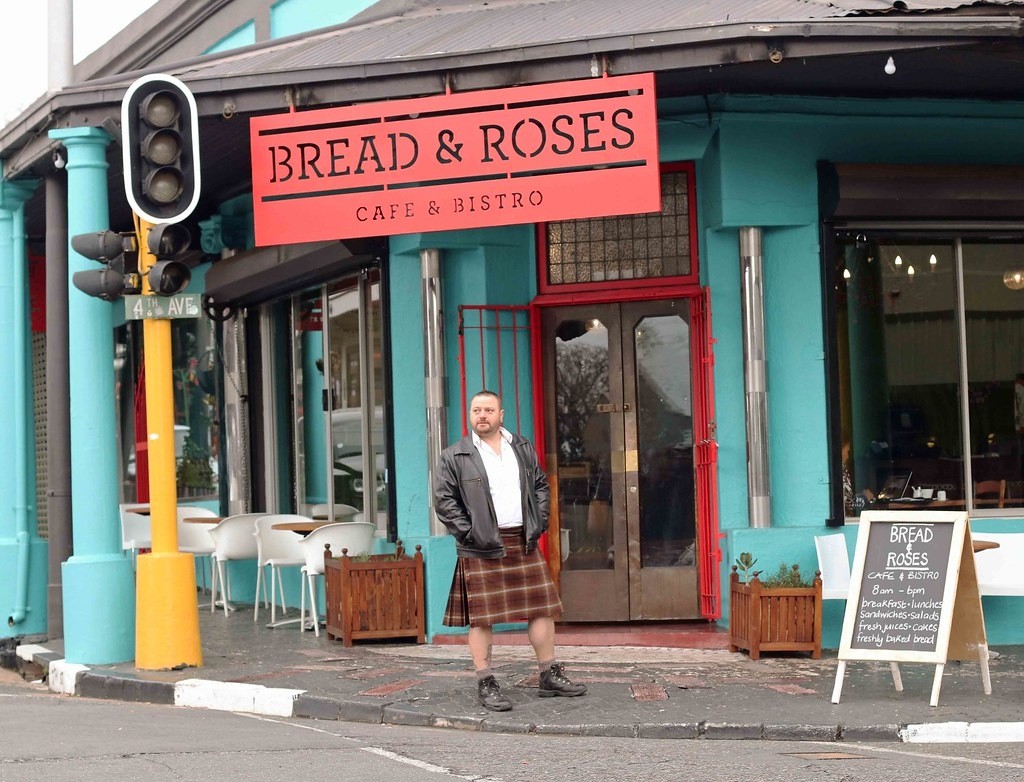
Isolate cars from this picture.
[126,426,220,482]
[326,406,387,499]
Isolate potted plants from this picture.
[324,538,426,646]
[730,552,823,660]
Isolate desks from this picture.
[865,500,1024,509]
[269,522,357,630]
[957,540,1000,664]
[183,518,263,611]
[127,508,150,517]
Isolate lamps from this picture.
[892,254,938,289]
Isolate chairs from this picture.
[119,503,376,637]
[312,503,387,536]
[973,479,1005,509]
[813,534,850,614]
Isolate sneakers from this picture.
[538,663,587,697]
[477,675,512,713]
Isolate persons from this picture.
[432,390,586,710]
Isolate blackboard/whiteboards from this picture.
[834,509,969,662]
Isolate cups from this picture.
[913,490,922,497]
[937,491,946,501]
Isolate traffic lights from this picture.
[70,232,139,303]
[121,73,201,224]
[148,224,190,297]
